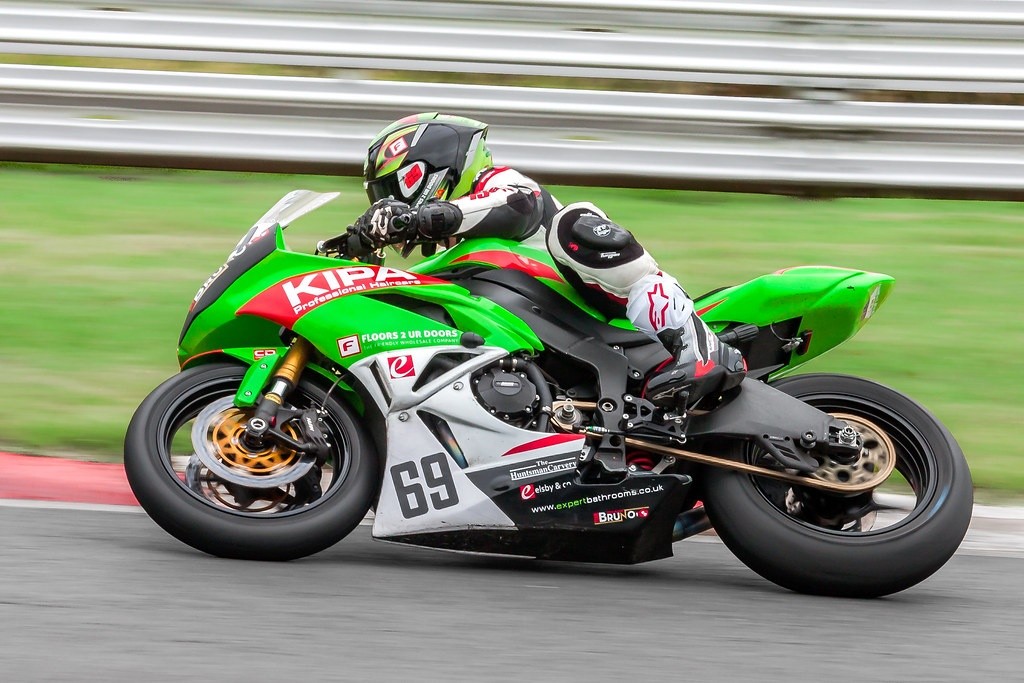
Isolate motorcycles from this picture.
[125,190,974,597]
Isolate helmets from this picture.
[362,112,494,259]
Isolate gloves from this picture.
[361,196,416,258]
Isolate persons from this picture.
[346,112,749,406]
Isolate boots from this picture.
[626,269,748,417]
